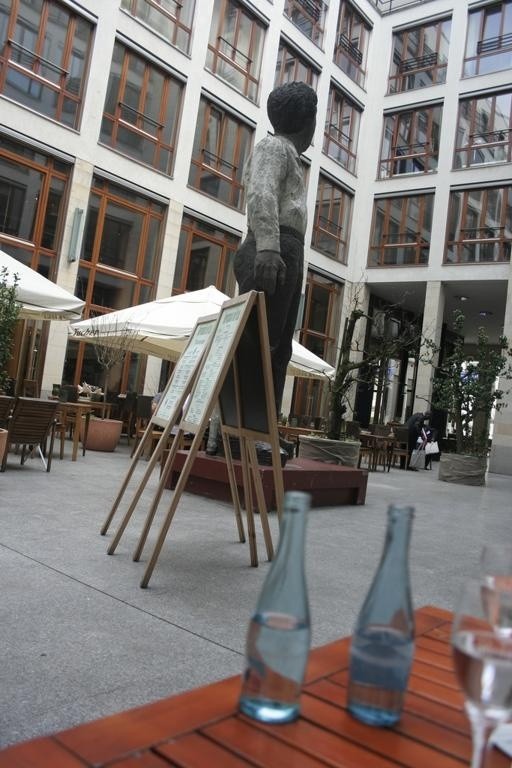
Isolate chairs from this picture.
[355,434,412,473]
[130,393,207,460]
[0,396,59,473]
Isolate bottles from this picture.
[237,490,310,724]
[346,504,416,728]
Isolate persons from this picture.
[150,384,170,461]
[399,409,433,471]
[231,80,318,469]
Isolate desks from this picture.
[77,390,115,419]
[53,399,92,461]
[1,602,512,768]
[277,423,326,458]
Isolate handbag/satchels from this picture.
[409,441,439,469]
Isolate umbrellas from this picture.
[67,284,337,383]
[0,248,88,322]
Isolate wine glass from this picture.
[448,546,512,768]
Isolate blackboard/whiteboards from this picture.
[152,290,258,434]
